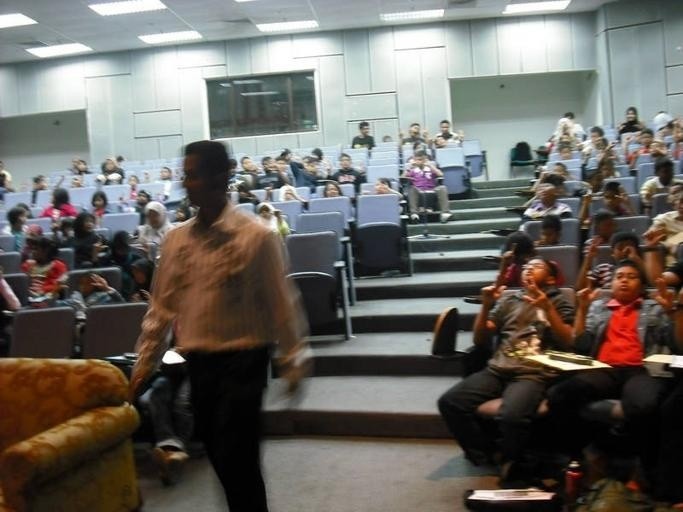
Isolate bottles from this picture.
[564,461,585,502]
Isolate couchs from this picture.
[0,358,143,511]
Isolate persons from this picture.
[0,118,465,512]
[432,107,683,512]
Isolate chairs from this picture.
[464,124,681,424]
[0,136,491,357]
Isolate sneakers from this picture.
[150,446,190,488]
[440,212,453,223]
[410,213,419,222]
